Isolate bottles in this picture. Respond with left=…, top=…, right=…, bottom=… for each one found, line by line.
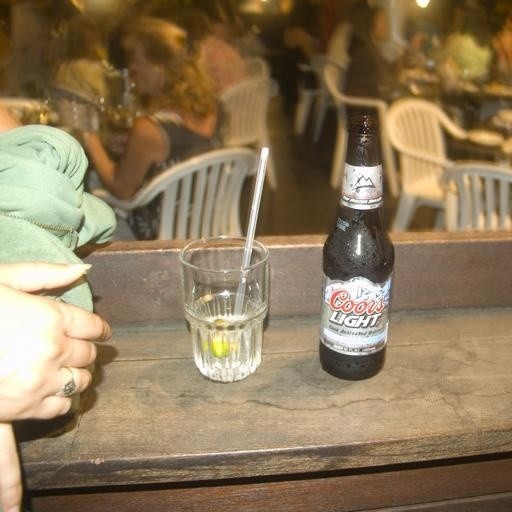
left=318, top=113, right=397, bottom=383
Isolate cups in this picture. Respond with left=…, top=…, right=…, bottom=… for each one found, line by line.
left=179, top=235, right=269, bottom=385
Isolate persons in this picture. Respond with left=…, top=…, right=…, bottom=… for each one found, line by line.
left=1, top=1, right=512, bottom=244
left=0, top=261, right=115, bottom=510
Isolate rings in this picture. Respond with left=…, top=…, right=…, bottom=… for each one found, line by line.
left=55, top=367, right=78, bottom=398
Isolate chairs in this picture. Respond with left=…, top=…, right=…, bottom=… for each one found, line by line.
left=1, top=96, right=54, bottom=126
left=380, top=96, right=479, bottom=241
left=439, top=162, right=510, bottom=239
left=89, top=145, right=266, bottom=239
left=292, top=20, right=510, bottom=189
left=214, top=56, right=283, bottom=191
